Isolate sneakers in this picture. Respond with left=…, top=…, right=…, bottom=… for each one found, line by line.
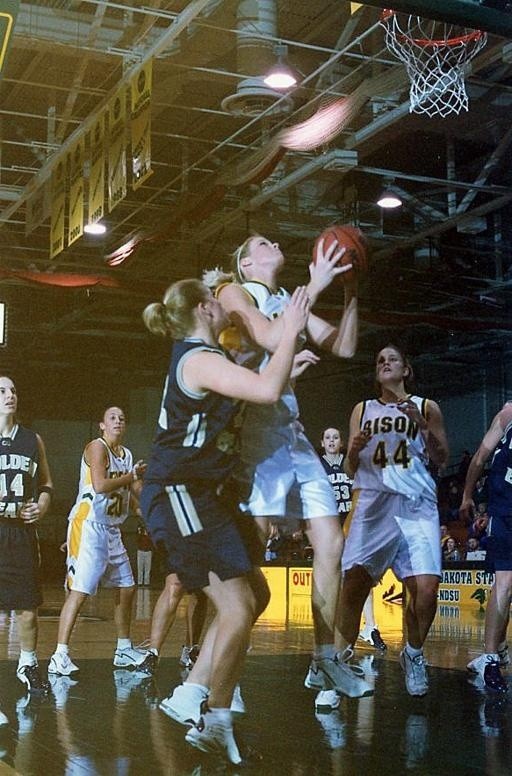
left=304, top=625, right=386, bottom=710
left=159, top=680, right=246, bottom=765
left=113, top=639, right=199, bottom=675
left=399, top=644, right=430, bottom=697
left=47, top=653, right=79, bottom=676
left=16, top=663, right=50, bottom=694
left=466, top=641, right=511, bottom=695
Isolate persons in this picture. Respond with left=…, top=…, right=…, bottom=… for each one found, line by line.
left=0, top=232, right=509, bottom=766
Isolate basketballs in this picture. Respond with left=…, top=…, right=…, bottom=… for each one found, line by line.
left=313, top=226, right=373, bottom=286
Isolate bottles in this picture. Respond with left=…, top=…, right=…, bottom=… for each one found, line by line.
left=454, top=549, right=459, bottom=561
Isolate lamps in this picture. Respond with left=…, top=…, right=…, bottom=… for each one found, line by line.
left=263, top=35, right=298, bottom=89
left=376, top=175, right=403, bottom=209
